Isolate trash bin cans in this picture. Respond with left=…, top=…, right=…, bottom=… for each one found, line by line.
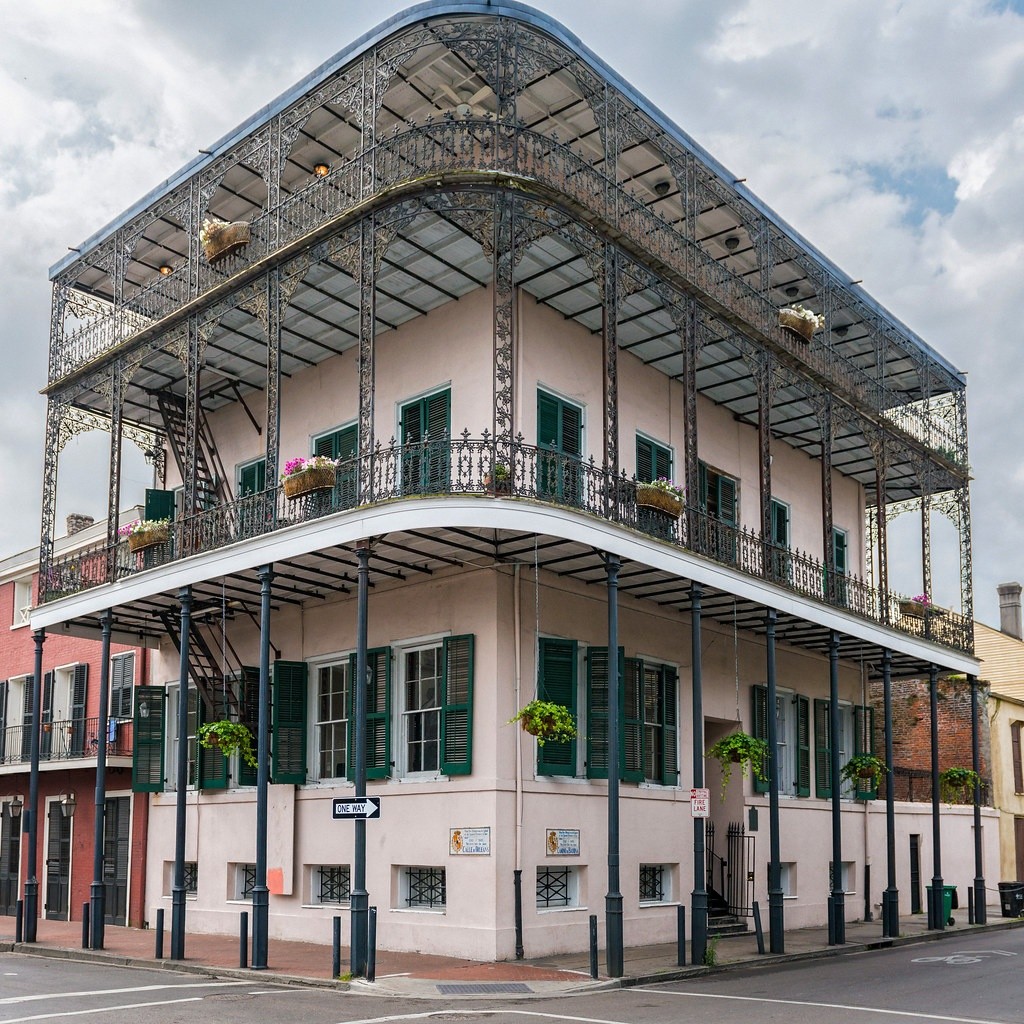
left=925, top=885, right=957, bottom=927
left=998, top=881, right=1024, bottom=917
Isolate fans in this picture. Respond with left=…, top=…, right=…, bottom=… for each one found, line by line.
left=430, top=82, right=496, bottom=119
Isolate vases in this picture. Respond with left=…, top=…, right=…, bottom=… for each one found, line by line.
left=203, top=221, right=250, bottom=265
left=899, top=600, right=926, bottom=619
left=283, top=468, right=337, bottom=499
left=127, top=528, right=167, bottom=553
left=779, top=308, right=813, bottom=344
left=636, top=484, right=682, bottom=519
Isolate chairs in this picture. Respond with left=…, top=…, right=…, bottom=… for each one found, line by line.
left=87, top=719, right=117, bottom=755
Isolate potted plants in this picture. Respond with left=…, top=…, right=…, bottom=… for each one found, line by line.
left=484, top=463, right=511, bottom=498
left=938, top=766, right=985, bottom=805
left=839, top=754, right=891, bottom=793
left=702, top=731, right=773, bottom=804
left=195, top=719, right=258, bottom=769
left=506, top=699, right=580, bottom=747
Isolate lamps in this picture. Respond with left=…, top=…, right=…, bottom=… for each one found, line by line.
left=159, top=265, right=173, bottom=275
left=649, top=177, right=850, bottom=339
left=313, top=163, right=330, bottom=177
left=6, top=789, right=24, bottom=818
left=59, top=787, right=78, bottom=818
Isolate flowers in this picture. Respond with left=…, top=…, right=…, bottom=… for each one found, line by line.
left=791, top=303, right=825, bottom=329
left=116, top=514, right=173, bottom=536
left=200, top=217, right=231, bottom=244
left=913, top=591, right=933, bottom=609
left=643, top=476, right=689, bottom=498
left=279, top=454, right=342, bottom=480
